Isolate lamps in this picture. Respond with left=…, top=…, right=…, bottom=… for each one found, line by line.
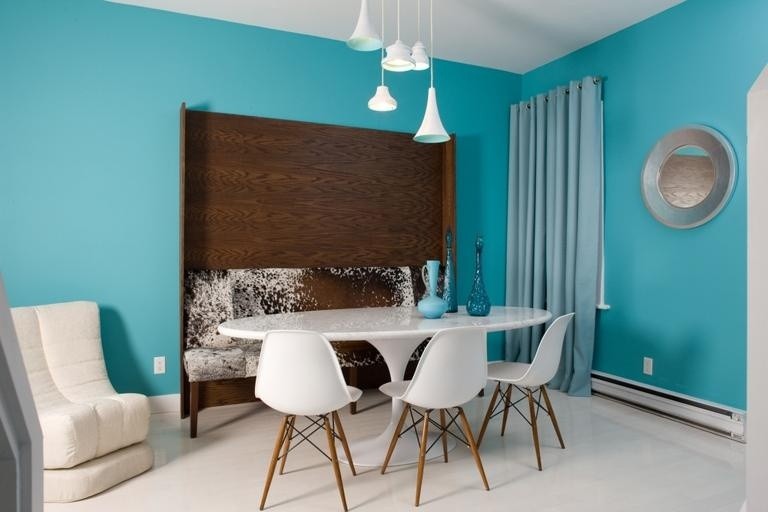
left=367, top=2, right=398, bottom=111
left=345, top=0, right=382, bottom=52
left=412, top=0, right=450, bottom=144
left=409, top=0, right=430, bottom=71
left=380, top=0, right=415, bottom=73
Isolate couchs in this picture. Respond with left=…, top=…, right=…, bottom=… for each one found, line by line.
left=1, top=298, right=155, bottom=505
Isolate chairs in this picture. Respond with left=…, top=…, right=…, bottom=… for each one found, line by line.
left=379, top=325, right=491, bottom=509
left=253, top=328, right=363, bottom=512
left=474, top=311, right=576, bottom=473
left=177, top=265, right=456, bottom=440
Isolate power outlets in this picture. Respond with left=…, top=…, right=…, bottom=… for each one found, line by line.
left=643, top=356, right=653, bottom=376
left=153, top=356, right=166, bottom=373
